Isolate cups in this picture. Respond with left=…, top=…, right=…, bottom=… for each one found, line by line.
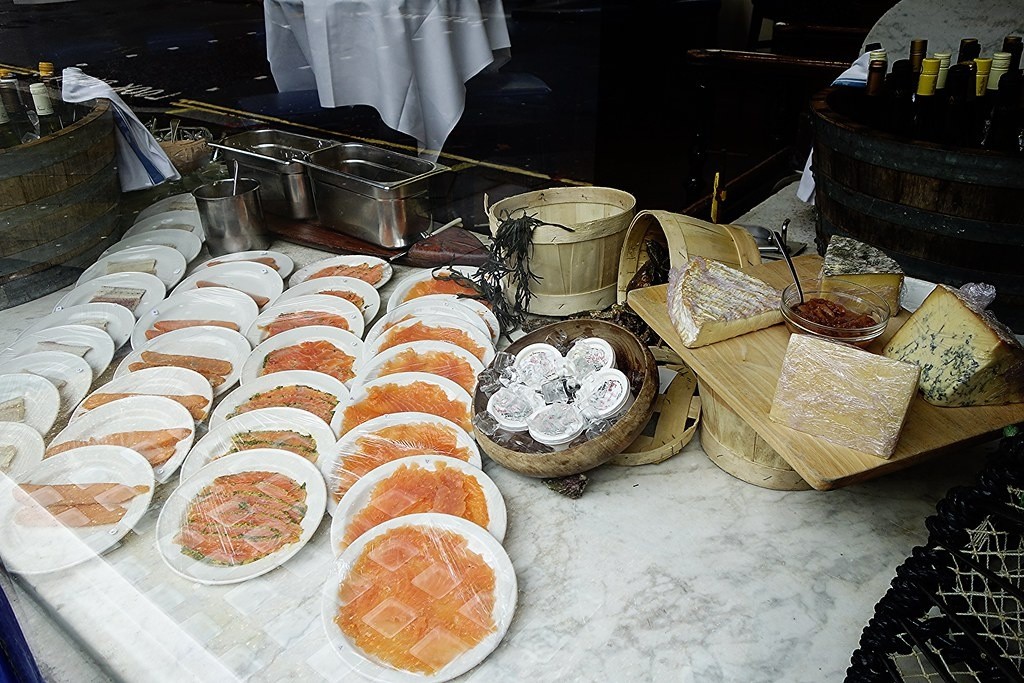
left=191, top=177, right=270, bottom=257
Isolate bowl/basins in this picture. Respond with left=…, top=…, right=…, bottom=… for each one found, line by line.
left=779, top=278, right=891, bottom=348
left=470, top=319, right=659, bottom=476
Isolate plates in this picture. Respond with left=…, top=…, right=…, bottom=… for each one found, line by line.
left=0, top=193, right=518, bottom=683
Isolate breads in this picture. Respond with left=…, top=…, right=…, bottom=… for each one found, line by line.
left=768, top=333, right=922, bottom=459
left=882, top=285, right=1024, bottom=406
left=666, top=255, right=786, bottom=348
left=819, top=235, right=904, bottom=316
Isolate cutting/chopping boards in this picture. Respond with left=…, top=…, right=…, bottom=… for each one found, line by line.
left=627, top=250, right=1024, bottom=490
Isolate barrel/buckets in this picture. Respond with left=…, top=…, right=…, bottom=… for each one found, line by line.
left=692, top=371, right=806, bottom=490
left=489, top=186, right=637, bottom=317
left=811, top=87, right=1024, bottom=330
left=617, top=209, right=762, bottom=303
left=0, top=98, right=135, bottom=311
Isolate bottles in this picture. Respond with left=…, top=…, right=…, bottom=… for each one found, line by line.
left=849, top=35, right=1024, bottom=158
left=0, top=62, right=65, bottom=148
